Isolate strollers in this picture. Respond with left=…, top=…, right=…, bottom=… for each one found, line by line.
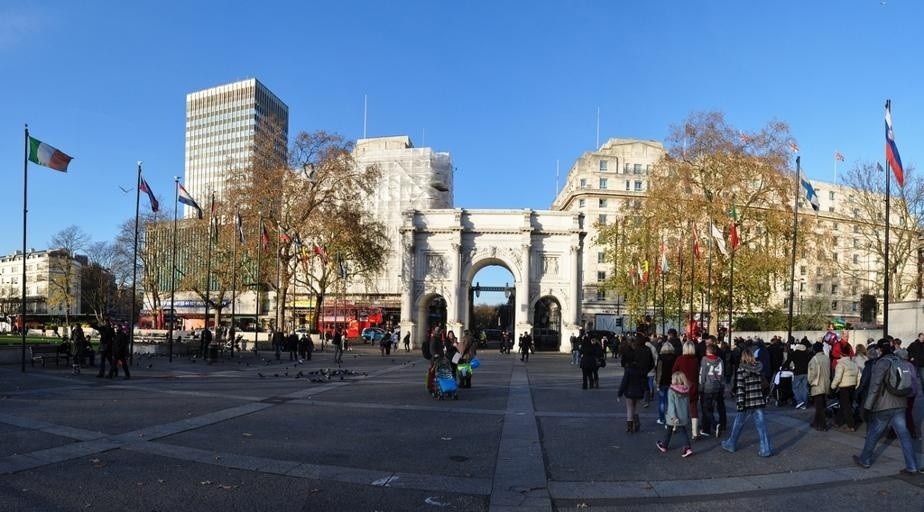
left=765, top=357, right=794, bottom=408
left=432, top=358, right=458, bottom=401
left=825, top=397, right=863, bottom=428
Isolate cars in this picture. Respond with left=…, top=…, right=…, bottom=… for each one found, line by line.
left=586, top=329, right=620, bottom=346
left=474, top=327, right=513, bottom=342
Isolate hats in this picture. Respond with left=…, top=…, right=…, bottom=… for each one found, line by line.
left=433, top=327, right=442, bottom=335
left=877, top=339, right=891, bottom=349
left=813, top=341, right=824, bottom=350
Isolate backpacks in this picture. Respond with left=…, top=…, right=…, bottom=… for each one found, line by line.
left=422, top=339, right=433, bottom=360
left=880, top=356, right=913, bottom=397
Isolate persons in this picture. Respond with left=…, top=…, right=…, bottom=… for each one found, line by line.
left=267, top=321, right=349, bottom=364
left=189, top=323, right=235, bottom=361
left=572, top=322, right=923, bottom=475
left=12, top=314, right=130, bottom=381
left=363, top=322, right=535, bottom=396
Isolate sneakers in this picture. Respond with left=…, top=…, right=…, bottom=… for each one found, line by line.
left=795, top=401, right=807, bottom=410
left=758, top=450, right=771, bottom=458
left=722, top=441, right=736, bottom=453
left=655, top=416, right=722, bottom=457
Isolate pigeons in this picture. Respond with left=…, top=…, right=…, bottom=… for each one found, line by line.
left=134, top=352, right=275, bottom=368
left=258, top=364, right=369, bottom=383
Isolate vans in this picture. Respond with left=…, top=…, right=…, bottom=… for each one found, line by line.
left=534, top=327, right=559, bottom=347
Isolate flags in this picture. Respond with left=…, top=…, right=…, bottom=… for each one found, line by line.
left=27, top=135, right=74, bottom=173
left=630, top=206, right=738, bottom=289
left=138, top=172, right=159, bottom=213
left=799, top=168, right=820, bottom=211
left=884, top=106, right=904, bottom=190
left=235, top=211, right=244, bottom=243
left=211, top=200, right=219, bottom=245
left=836, top=152, right=846, bottom=164
left=260, top=217, right=348, bottom=279
left=176, top=181, right=202, bottom=220
left=787, top=140, right=799, bottom=154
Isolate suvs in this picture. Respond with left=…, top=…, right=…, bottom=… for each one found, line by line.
left=685, top=312, right=734, bottom=341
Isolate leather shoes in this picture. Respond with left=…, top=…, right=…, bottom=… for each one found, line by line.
left=900, top=468, right=918, bottom=475
left=853, top=455, right=870, bottom=469
left=809, top=422, right=856, bottom=431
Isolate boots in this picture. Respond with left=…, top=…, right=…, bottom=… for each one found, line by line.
left=625, top=421, right=633, bottom=432
left=75, top=364, right=80, bottom=375
left=634, top=414, right=641, bottom=431
left=71, top=365, right=75, bottom=375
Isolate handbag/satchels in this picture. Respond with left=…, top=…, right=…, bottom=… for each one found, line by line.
left=437, top=376, right=457, bottom=393
left=451, top=352, right=462, bottom=364
left=808, top=358, right=820, bottom=387
left=715, top=385, right=727, bottom=400
left=600, top=359, right=606, bottom=367
left=760, top=373, right=770, bottom=395
left=825, top=397, right=840, bottom=414
left=458, top=357, right=464, bottom=364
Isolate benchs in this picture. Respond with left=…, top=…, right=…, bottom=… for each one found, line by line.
left=157, top=342, right=187, bottom=357
left=28, top=344, right=75, bottom=367
